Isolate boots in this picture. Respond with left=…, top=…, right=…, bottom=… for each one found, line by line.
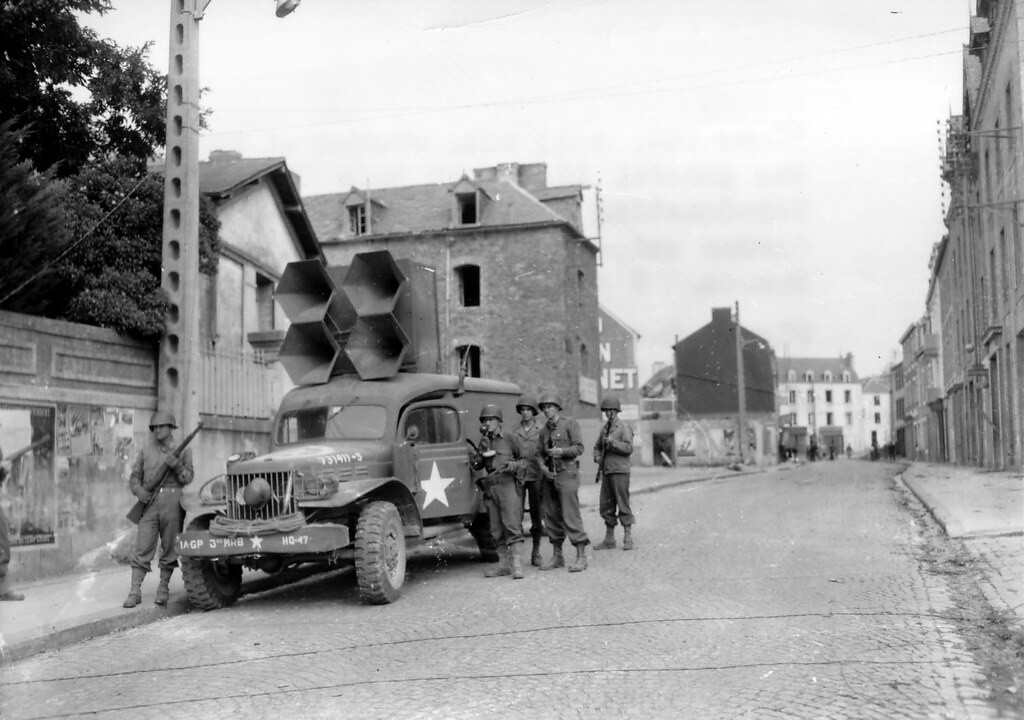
left=540, top=542, right=565, bottom=570
left=592, top=525, right=617, bottom=550
left=624, top=524, right=634, bottom=550
left=568, top=543, right=588, bottom=572
left=485, top=544, right=512, bottom=576
left=510, top=540, right=524, bottom=579
left=531, top=534, right=543, bottom=566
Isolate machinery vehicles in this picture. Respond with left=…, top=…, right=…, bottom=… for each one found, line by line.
left=173, top=249, right=527, bottom=611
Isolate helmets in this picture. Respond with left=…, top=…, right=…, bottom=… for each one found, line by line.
left=148, top=411, right=177, bottom=431
left=516, top=397, right=540, bottom=416
left=601, top=396, right=621, bottom=412
left=479, top=405, right=504, bottom=424
left=244, top=478, right=271, bottom=511
left=538, top=394, right=563, bottom=412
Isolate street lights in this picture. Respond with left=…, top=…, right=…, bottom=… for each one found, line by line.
left=156, top=0, right=304, bottom=506
left=735, top=300, right=766, bottom=463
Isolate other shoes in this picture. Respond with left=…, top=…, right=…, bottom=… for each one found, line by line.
left=154, top=585, right=169, bottom=603
left=0, top=590, right=25, bottom=601
left=122, top=584, right=141, bottom=607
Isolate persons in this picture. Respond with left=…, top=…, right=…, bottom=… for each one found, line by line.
left=0, top=449, right=24, bottom=602
left=507, top=395, right=591, bottom=571
left=593, top=395, right=635, bottom=550
left=474, top=402, right=527, bottom=580
left=122, top=411, right=194, bottom=608
left=777, top=441, right=898, bottom=462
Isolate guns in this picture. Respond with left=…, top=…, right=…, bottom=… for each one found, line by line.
left=596, top=411, right=614, bottom=483
left=126, top=419, right=205, bottom=526
left=488, top=454, right=516, bottom=477
left=546, top=436, right=563, bottom=508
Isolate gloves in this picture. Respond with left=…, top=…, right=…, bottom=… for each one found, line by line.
left=166, top=454, right=183, bottom=471
left=136, top=487, right=152, bottom=504
left=503, top=461, right=517, bottom=473
left=543, top=466, right=558, bottom=480
left=548, top=447, right=563, bottom=458
